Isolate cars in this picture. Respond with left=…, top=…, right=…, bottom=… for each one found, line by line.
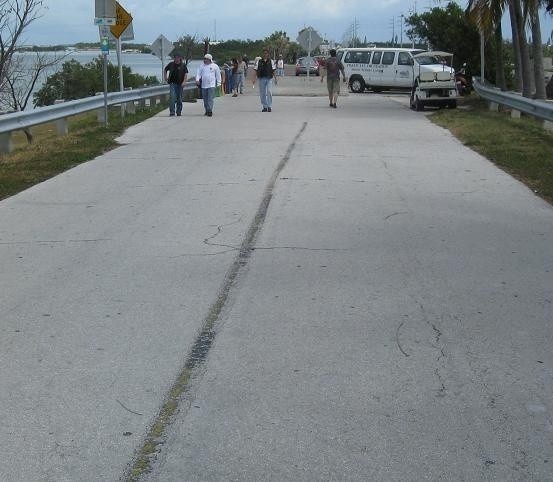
left=408, top=50, right=458, bottom=111
left=295, top=56, right=320, bottom=76
left=314, top=55, right=326, bottom=66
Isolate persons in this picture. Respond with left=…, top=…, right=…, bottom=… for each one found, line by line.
left=163, top=53, right=189, bottom=117
left=249, top=49, right=277, bottom=113
left=319, top=49, right=346, bottom=109
left=222, top=53, right=262, bottom=97
left=269, top=54, right=285, bottom=81
left=194, top=54, right=221, bottom=117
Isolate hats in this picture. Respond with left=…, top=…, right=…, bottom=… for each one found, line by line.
left=204, top=54, right=212, bottom=61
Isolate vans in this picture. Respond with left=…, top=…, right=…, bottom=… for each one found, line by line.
left=335, top=47, right=426, bottom=93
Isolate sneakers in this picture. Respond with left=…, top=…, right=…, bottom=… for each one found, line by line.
left=329, top=103, right=336, bottom=108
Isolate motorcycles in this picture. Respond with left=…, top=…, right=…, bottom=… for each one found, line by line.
left=453, top=62, right=472, bottom=96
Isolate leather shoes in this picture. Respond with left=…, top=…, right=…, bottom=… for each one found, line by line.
left=170, top=113, right=181, bottom=116
left=262, top=107, right=271, bottom=112
left=205, top=111, right=212, bottom=116
